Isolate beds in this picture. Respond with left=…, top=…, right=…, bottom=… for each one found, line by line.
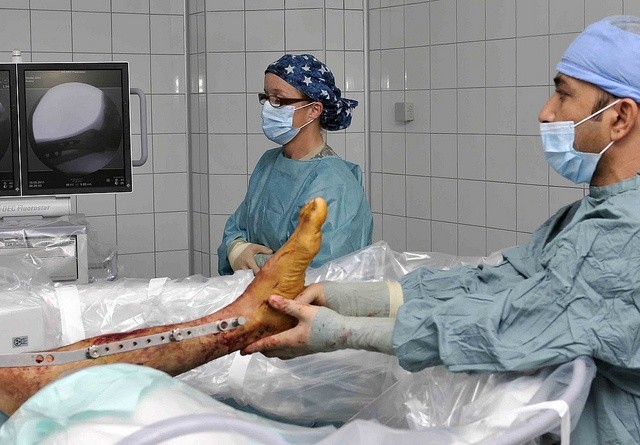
left=2, top=248, right=595, bottom=445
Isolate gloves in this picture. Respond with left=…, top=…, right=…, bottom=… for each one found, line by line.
left=240, top=294, right=396, bottom=360
left=293, top=279, right=404, bottom=319
left=226, top=237, right=273, bottom=276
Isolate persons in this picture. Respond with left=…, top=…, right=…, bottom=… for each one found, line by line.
left=0, top=197, right=327, bottom=417
left=217, top=53, right=373, bottom=279
left=241, top=15, right=640, bottom=444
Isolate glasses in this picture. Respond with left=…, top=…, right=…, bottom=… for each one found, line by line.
left=257, top=91, right=310, bottom=107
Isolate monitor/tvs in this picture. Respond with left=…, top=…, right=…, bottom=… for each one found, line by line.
left=17, top=60, right=134, bottom=198
left=0, top=62, right=21, bottom=199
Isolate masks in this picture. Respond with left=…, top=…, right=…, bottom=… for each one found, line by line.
left=260, top=99, right=320, bottom=145
left=538, top=99, right=623, bottom=185
